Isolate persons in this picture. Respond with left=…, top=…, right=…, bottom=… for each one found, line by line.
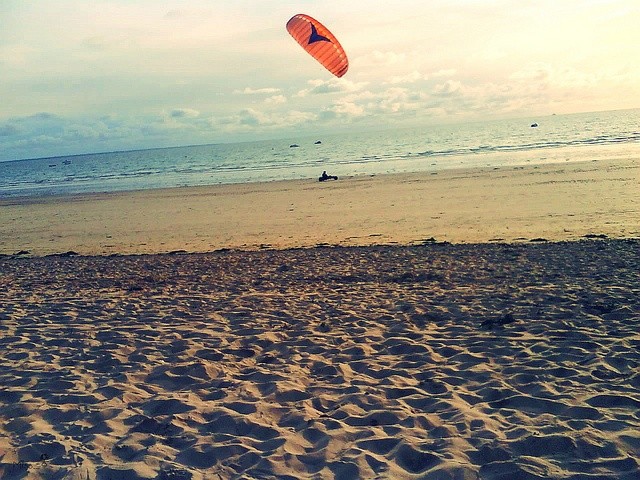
left=322, top=170, right=328, bottom=177
